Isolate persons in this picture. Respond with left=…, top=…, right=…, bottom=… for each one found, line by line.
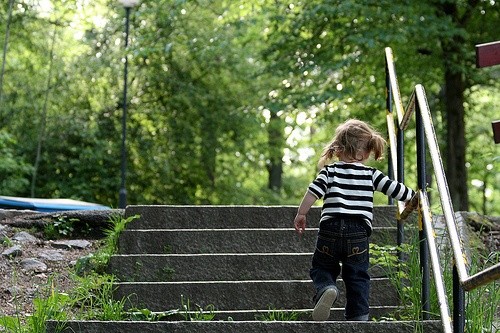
left=294, top=119, right=419, bottom=321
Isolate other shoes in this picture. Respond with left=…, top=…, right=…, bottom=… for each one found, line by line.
left=312, top=288, right=337, bottom=320
